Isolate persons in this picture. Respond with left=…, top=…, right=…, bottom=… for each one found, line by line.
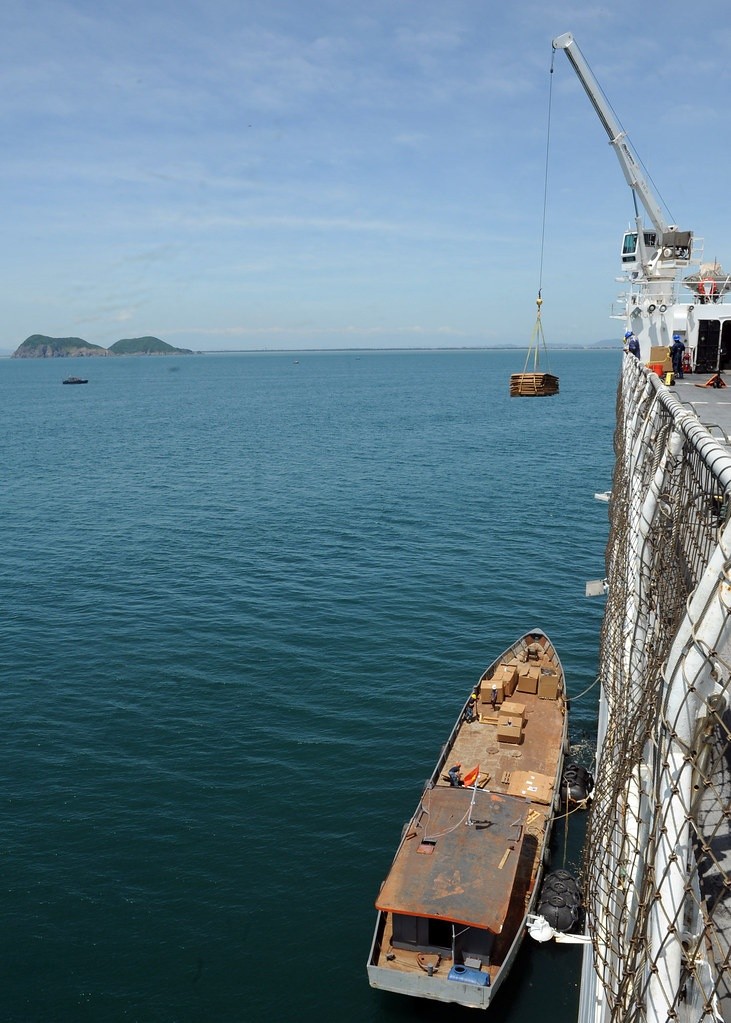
left=669, top=334, right=685, bottom=379
left=448, top=763, right=462, bottom=787
left=623, top=331, right=641, bottom=361
left=465, top=694, right=477, bottom=723
left=489, top=685, right=498, bottom=709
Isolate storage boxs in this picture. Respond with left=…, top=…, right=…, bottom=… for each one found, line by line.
left=480, top=680, right=504, bottom=704
left=650, top=346, right=673, bottom=372
left=496, top=716, right=523, bottom=745
left=491, top=672, right=515, bottom=697
left=498, top=701, right=526, bottom=724
left=518, top=665, right=539, bottom=693
left=538, top=667, right=560, bottom=700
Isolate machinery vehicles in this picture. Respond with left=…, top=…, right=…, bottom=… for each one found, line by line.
left=547, top=30, right=693, bottom=286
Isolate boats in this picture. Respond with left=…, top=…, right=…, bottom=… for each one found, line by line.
left=363, top=625, right=568, bottom=1012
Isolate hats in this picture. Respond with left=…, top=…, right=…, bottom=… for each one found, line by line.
left=492, top=684, right=497, bottom=689
left=472, top=694, right=476, bottom=699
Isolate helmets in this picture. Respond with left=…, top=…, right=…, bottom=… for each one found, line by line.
left=674, top=335, right=680, bottom=341
left=625, top=332, right=633, bottom=338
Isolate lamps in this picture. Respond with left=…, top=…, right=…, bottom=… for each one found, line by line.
left=594, top=491, right=612, bottom=501
left=585, top=578, right=609, bottom=598
